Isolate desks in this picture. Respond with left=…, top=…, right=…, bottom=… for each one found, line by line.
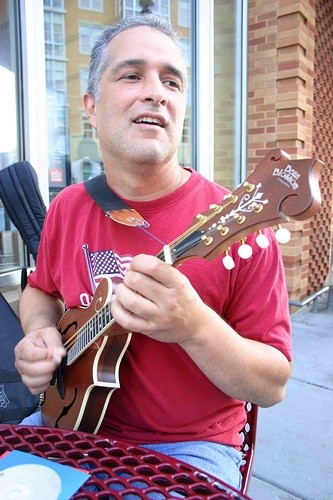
left=0, top=423, right=251, bottom=500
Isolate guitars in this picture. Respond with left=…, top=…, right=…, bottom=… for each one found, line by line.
left=37, top=148, right=325, bottom=435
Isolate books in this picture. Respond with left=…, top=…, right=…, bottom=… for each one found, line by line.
left=0, top=450, right=91, bottom=500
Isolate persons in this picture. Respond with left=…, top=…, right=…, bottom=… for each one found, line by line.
left=0, top=17, right=294, bottom=500
